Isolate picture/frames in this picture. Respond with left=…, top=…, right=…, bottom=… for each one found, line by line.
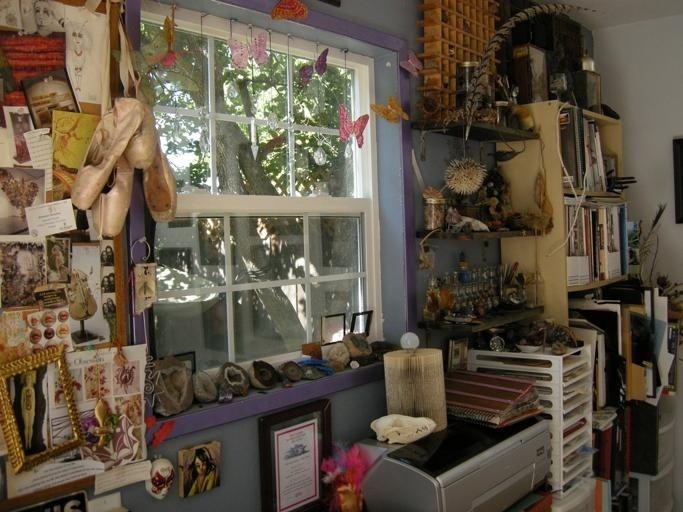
left=0, top=344, right=84, bottom=476
left=256, top=397, right=333, bottom=512
left=158, top=350, right=197, bottom=376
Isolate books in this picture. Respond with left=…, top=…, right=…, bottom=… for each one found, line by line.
left=559, top=107, right=633, bottom=288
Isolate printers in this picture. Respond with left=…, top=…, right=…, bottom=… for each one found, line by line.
left=355, top=415, right=552, bottom=512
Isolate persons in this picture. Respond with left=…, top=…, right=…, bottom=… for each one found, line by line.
left=188, top=448, right=217, bottom=497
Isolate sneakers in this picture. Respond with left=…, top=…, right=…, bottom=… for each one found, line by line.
left=69, top=68, right=176, bottom=240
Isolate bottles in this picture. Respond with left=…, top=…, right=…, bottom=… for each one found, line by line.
left=422, top=196, right=447, bottom=231
left=491, top=100, right=509, bottom=129
left=455, top=59, right=480, bottom=91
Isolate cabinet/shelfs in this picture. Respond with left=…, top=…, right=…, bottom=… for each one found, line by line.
left=409, top=119, right=546, bottom=335
left=467, top=343, right=594, bottom=500
left=494, top=99, right=629, bottom=342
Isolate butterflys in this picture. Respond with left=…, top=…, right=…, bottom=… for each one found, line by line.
left=337, top=104, right=369, bottom=149
left=228, top=30, right=269, bottom=71
left=298, top=47, right=330, bottom=87
left=369, top=96, right=412, bottom=124
left=397, top=48, right=424, bottom=76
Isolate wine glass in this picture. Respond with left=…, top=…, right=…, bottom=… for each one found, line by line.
left=548, top=72, right=570, bottom=103
